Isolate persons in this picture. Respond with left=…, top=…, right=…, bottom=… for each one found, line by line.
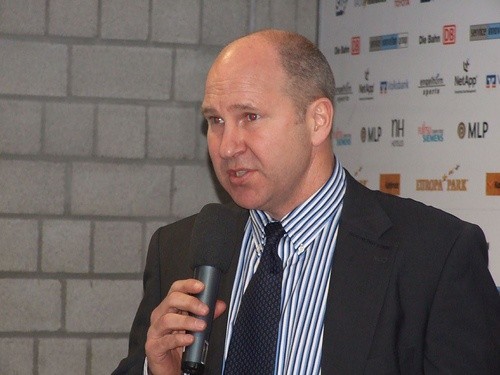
left=105, top=28, right=499, bottom=374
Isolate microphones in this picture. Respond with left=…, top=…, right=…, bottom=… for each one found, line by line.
left=180, top=202, right=240, bottom=375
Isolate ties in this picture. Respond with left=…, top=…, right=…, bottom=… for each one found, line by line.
left=222, top=222, right=287, bottom=375
